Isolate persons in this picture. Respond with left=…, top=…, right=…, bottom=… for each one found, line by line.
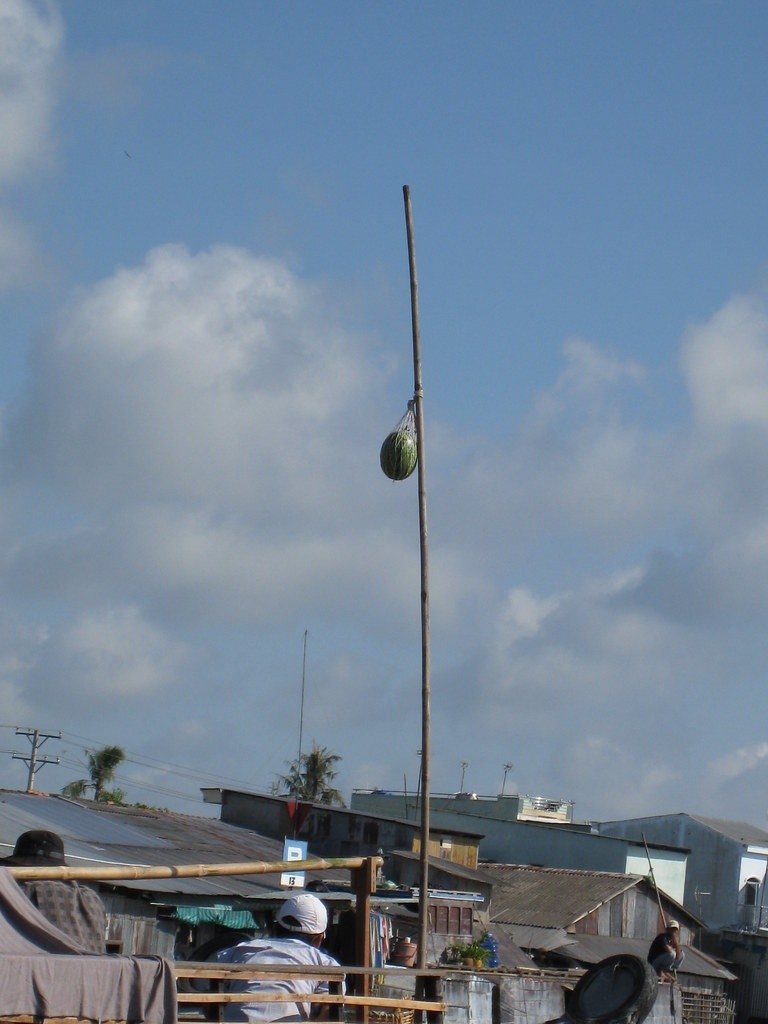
left=646, top=919, right=685, bottom=982
left=197, top=894, right=348, bottom=1022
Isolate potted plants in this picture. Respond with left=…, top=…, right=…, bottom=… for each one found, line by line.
left=446, top=928, right=492, bottom=972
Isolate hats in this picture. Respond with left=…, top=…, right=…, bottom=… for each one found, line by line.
left=666, top=920, right=679, bottom=931
left=0, top=830, right=67, bottom=866
left=275, top=894, right=328, bottom=939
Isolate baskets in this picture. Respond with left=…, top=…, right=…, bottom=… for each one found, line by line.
left=348, top=994, right=414, bottom=1024
